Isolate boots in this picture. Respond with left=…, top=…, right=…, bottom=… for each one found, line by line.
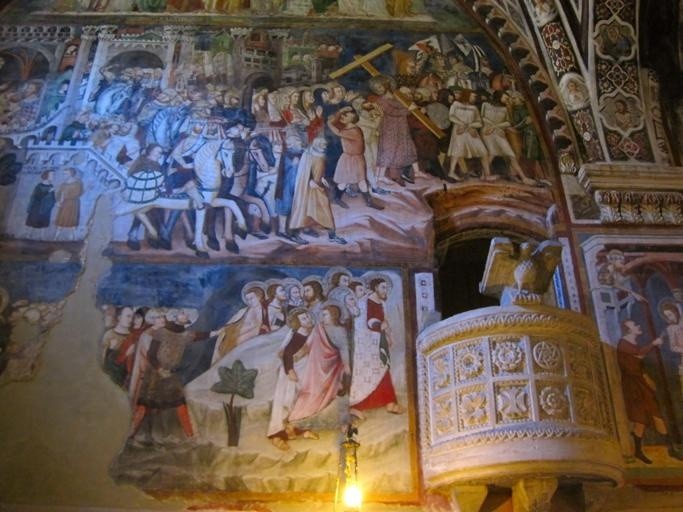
left=362, top=191, right=386, bottom=211
left=631, top=433, right=654, bottom=464
left=333, top=188, right=351, bottom=209
left=659, top=434, right=683, bottom=461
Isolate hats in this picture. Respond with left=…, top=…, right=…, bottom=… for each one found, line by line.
left=417, top=87, right=431, bottom=100
left=310, top=137, right=327, bottom=155
left=126, top=306, right=199, bottom=329
left=241, top=267, right=394, bottom=328
left=391, top=50, right=408, bottom=66
left=210, top=82, right=346, bottom=103
left=491, top=76, right=501, bottom=89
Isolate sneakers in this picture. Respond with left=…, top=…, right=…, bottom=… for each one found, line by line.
left=304, top=228, right=319, bottom=238
left=328, top=231, right=347, bottom=245
left=270, top=401, right=407, bottom=452
left=447, top=169, right=553, bottom=190
left=373, top=173, right=432, bottom=196
